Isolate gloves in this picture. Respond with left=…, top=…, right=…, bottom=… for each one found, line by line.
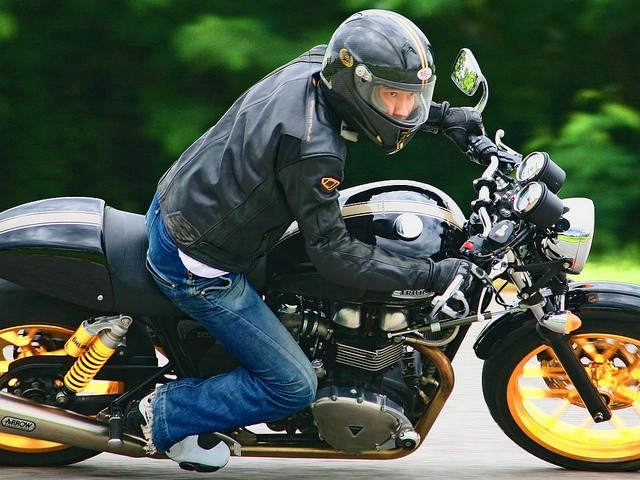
left=428, top=257, right=472, bottom=298
left=440, top=102, right=485, bottom=153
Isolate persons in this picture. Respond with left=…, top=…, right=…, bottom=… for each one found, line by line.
left=138, top=10, right=492, bottom=474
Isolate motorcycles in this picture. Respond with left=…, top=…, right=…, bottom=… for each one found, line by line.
left=1, top=44, right=639, bottom=474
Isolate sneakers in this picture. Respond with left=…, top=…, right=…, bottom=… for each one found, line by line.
left=124, top=395, right=230, bottom=472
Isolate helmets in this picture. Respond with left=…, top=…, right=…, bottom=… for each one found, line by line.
left=319, top=9, right=436, bottom=156
